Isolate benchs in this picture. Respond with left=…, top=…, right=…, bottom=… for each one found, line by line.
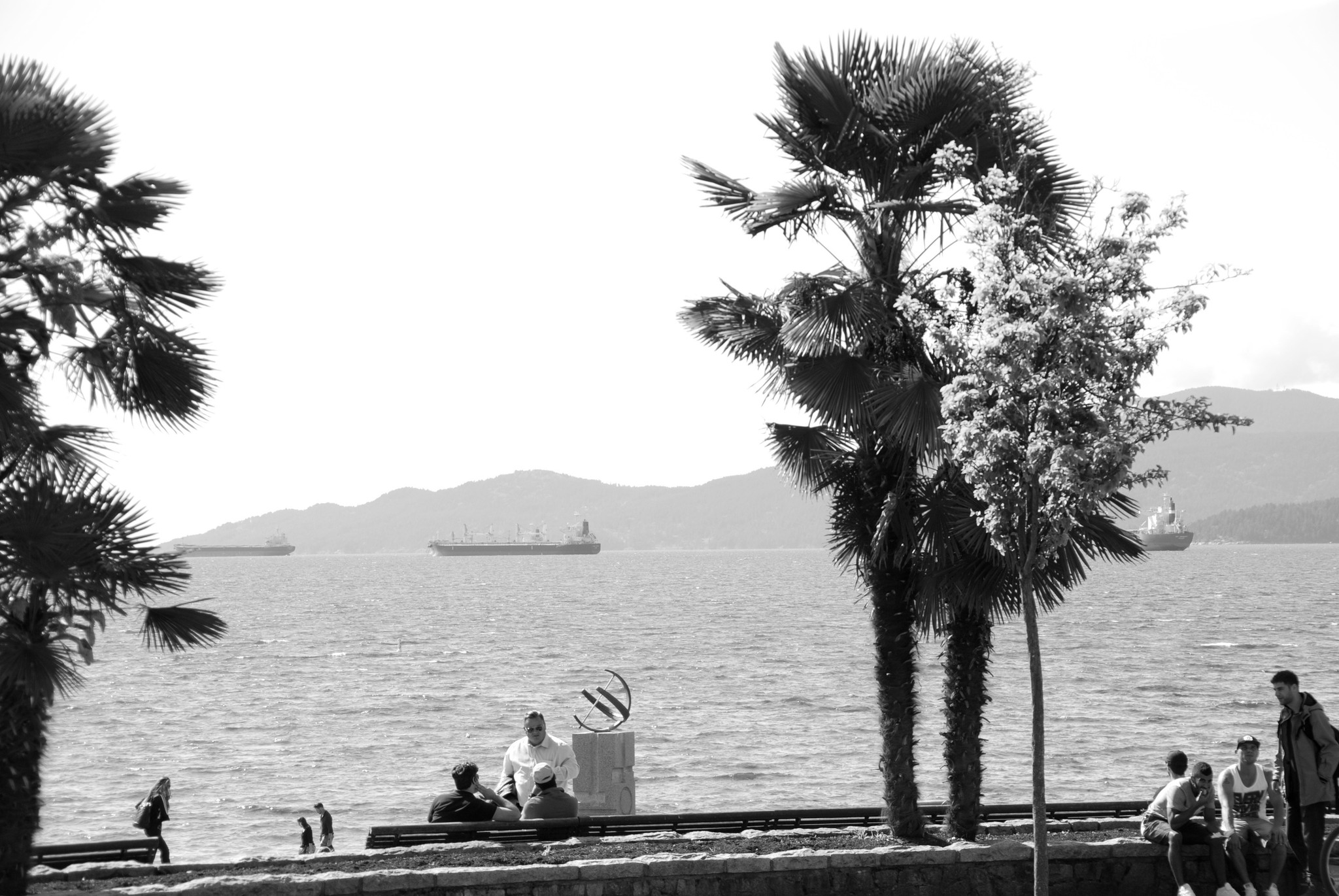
left=26, top=835, right=158, bottom=871
left=365, top=799, right=1157, bottom=838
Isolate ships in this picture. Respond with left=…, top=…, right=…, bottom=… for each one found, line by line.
left=171, top=532, right=295, bottom=556
left=1124, top=492, right=1194, bottom=552
left=429, top=520, right=600, bottom=556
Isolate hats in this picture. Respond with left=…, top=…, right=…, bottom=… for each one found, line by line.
left=1237, top=735, right=1260, bottom=751
left=1165, top=751, right=1187, bottom=766
left=533, top=762, right=554, bottom=784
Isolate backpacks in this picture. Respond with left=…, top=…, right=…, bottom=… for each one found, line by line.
left=133, top=796, right=154, bottom=829
left=1294, top=714, right=1339, bottom=798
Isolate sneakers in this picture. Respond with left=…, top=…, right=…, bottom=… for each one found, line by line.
left=1216, top=882, right=1241, bottom=896
left=1266, top=883, right=1279, bottom=896
left=1243, top=882, right=1257, bottom=896
left=1177, top=883, right=1196, bottom=896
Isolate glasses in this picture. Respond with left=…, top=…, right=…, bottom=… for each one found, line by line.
left=525, top=725, right=544, bottom=733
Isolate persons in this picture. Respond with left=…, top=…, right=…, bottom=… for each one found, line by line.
left=1270, top=669, right=1339, bottom=896
left=135, top=776, right=172, bottom=865
left=499, top=708, right=579, bottom=814
left=520, top=761, right=580, bottom=825
left=296, top=816, right=316, bottom=854
left=313, top=801, right=338, bottom=853
left=1140, top=762, right=1242, bottom=896
left=1215, top=733, right=1290, bottom=896
left=426, top=760, right=522, bottom=841
left=316, top=846, right=332, bottom=854
left=1152, top=749, right=1190, bottom=802
